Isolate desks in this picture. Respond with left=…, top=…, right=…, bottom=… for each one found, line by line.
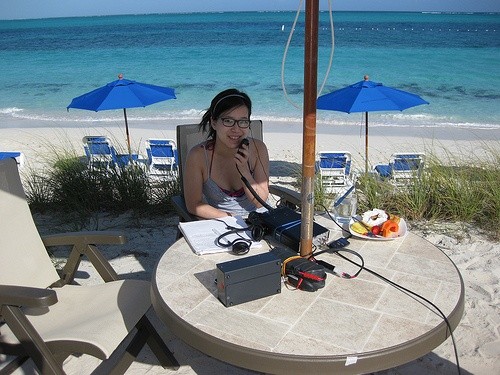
left=150, top=217, right=465, bottom=375
left=118, top=153, right=139, bottom=165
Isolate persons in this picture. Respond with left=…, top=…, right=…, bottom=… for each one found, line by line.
left=183, top=89, right=272, bottom=219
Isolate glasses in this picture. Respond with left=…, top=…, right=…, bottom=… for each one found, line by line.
left=216, top=117, right=251, bottom=128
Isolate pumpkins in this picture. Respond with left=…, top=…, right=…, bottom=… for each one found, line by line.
left=381, top=220, right=398, bottom=237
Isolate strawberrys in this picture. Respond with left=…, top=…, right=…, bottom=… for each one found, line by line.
left=372, top=226, right=380, bottom=235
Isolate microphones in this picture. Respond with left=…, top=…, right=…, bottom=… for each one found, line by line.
left=236, top=139, right=249, bottom=163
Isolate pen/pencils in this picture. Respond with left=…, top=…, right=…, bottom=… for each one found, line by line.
left=213, top=228, right=232, bottom=245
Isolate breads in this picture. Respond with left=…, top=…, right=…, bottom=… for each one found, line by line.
left=351, top=223, right=369, bottom=234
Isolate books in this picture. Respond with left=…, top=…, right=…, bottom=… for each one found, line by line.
left=178, top=215, right=262, bottom=255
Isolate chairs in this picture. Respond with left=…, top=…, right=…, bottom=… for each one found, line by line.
left=316, top=150, right=354, bottom=189
left=145, top=138, right=179, bottom=175
left=169, top=120, right=304, bottom=243
left=0, top=154, right=183, bottom=375
left=374, top=151, right=429, bottom=188
left=81, top=135, right=123, bottom=173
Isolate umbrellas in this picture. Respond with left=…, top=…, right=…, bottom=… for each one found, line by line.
left=317, top=75, right=430, bottom=173
left=66, top=74, right=177, bottom=165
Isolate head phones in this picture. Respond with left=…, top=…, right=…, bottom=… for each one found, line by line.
left=218, top=222, right=264, bottom=254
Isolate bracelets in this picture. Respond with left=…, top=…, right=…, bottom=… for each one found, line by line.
left=226, top=212, right=232, bottom=217
